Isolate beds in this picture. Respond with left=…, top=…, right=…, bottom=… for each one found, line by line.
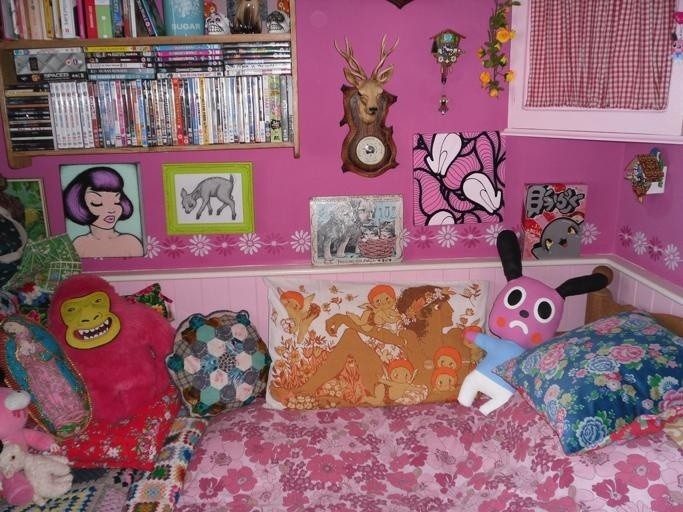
left=0, top=266, right=683, bottom=512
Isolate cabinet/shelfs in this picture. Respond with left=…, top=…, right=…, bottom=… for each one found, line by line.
left=1, top=0, right=300, bottom=169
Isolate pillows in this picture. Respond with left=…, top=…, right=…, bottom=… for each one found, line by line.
left=262, top=279, right=490, bottom=413
left=494, top=307, right=683, bottom=454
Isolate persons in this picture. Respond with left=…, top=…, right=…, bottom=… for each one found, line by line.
left=285, top=285, right=471, bottom=404
left=65, top=166, right=143, bottom=256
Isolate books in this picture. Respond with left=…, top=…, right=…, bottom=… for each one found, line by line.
left=1, top=1, right=296, bottom=151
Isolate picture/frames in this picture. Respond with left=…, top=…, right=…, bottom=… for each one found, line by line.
left=310, top=194, right=405, bottom=265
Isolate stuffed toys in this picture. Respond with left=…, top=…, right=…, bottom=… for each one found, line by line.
left=0, top=274, right=177, bottom=507
left=0, top=273, right=176, bottom=506
left=457, top=229, right=608, bottom=415
